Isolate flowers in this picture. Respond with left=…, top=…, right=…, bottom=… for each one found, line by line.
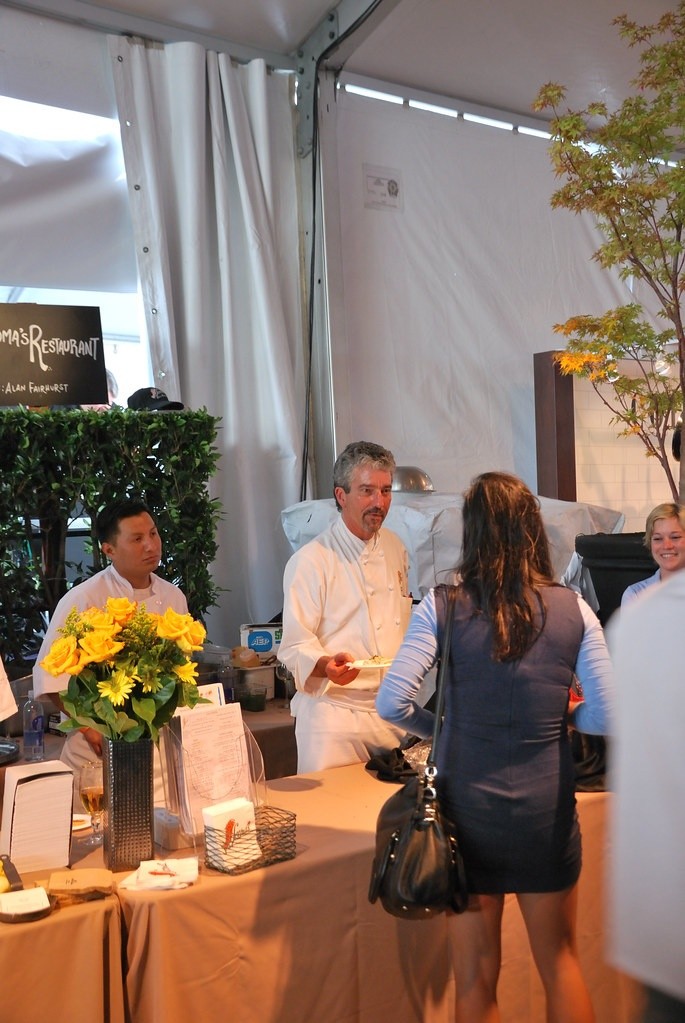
left=39, top=600, right=210, bottom=745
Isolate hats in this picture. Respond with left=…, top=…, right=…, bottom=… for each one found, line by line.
left=128, top=387, right=184, bottom=411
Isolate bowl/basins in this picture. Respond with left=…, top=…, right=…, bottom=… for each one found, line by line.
left=390, top=466, right=436, bottom=493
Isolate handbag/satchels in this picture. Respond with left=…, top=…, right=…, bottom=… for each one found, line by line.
left=368, top=768, right=468, bottom=921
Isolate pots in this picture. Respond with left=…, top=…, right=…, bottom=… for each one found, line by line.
left=1, top=853, right=57, bottom=924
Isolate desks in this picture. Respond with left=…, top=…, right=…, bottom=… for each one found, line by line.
left=0, top=868, right=123, bottom=1023
left=70, top=760, right=645, bottom=1023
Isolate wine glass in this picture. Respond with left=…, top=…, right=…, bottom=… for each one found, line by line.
left=276, top=658, right=294, bottom=710
left=78, top=761, right=106, bottom=846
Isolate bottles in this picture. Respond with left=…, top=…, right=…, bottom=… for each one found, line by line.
left=22, top=690, right=46, bottom=761
left=217, top=655, right=235, bottom=700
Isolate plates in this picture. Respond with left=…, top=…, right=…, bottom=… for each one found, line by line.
left=345, top=660, right=393, bottom=670
left=72, top=813, right=92, bottom=831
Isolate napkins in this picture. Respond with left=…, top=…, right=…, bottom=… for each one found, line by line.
left=122, top=797, right=262, bottom=891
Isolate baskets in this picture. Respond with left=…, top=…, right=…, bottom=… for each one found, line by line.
left=204, top=806, right=297, bottom=876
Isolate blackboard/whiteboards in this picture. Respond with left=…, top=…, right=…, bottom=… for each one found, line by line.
left=0, top=302, right=109, bottom=406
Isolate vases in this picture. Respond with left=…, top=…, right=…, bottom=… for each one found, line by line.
left=103, top=732, right=157, bottom=872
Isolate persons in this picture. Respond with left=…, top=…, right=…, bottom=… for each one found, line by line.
left=612, top=569, right=685, bottom=1023
left=376, top=471, right=612, bottom=1023
left=622, top=503, right=685, bottom=604
left=32, top=499, right=185, bottom=816
left=277, top=441, right=417, bottom=774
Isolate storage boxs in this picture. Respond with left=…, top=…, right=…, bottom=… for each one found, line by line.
left=240, top=621, right=282, bottom=655
